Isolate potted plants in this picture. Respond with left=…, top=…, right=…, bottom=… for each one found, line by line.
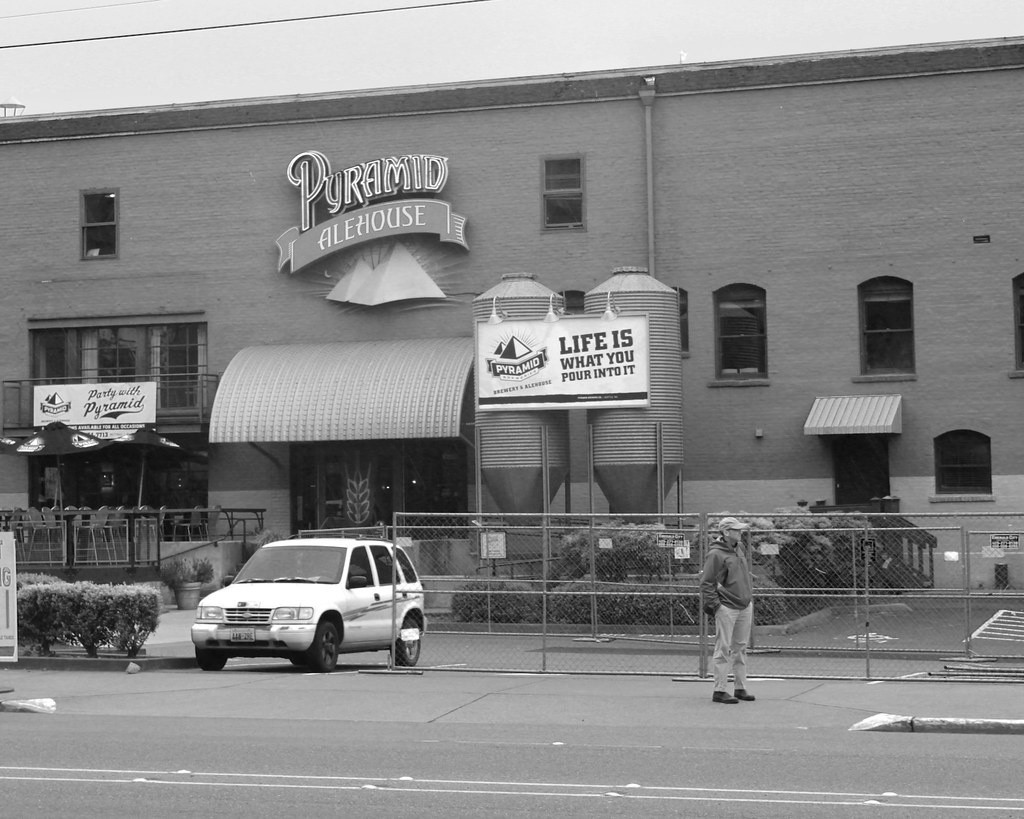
left=159, top=555, right=215, bottom=610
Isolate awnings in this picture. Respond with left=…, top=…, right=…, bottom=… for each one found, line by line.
left=804, top=394, right=902, bottom=435
left=208, top=337, right=475, bottom=443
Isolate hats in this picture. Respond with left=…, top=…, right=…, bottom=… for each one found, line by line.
left=718, top=517, right=748, bottom=531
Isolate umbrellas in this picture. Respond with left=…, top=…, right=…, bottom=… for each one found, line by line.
left=109, top=427, right=188, bottom=508
left=0, top=422, right=114, bottom=519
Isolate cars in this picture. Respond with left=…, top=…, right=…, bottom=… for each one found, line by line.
left=188, top=539, right=427, bottom=670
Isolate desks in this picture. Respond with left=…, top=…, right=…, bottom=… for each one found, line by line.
left=0, top=507, right=267, bottom=565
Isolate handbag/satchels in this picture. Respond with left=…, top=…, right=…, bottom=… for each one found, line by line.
left=703, top=602, right=715, bottom=616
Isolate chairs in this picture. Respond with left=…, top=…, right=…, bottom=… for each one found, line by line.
left=1, top=502, right=223, bottom=566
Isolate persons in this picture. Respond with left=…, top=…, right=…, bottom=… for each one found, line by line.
left=699, top=517, right=755, bottom=704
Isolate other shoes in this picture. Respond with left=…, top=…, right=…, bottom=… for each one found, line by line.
left=712, top=691, right=738, bottom=703
left=734, top=689, right=755, bottom=700
